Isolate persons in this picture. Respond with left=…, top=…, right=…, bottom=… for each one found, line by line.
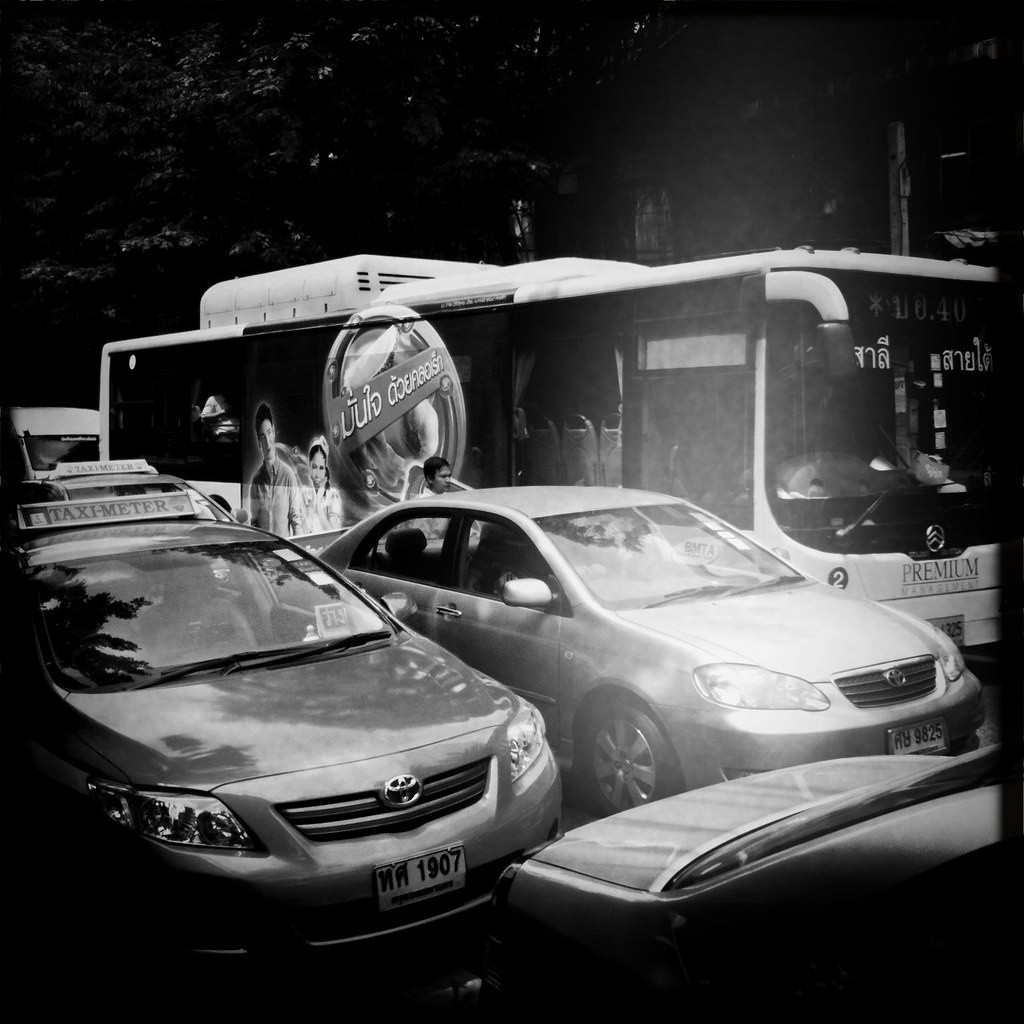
left=412, top=457, right=453, bottom=539
left=300, top=434, right=343, bottom=535
left=250, top=403, right=303, bottom=538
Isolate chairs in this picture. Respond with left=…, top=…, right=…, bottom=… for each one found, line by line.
left=383, top=523, right=693, bottom=606
left=523, top=410, right=622, bottom=495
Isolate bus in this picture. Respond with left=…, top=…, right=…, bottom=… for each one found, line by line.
left=100, top=244, right=1007, bottom=654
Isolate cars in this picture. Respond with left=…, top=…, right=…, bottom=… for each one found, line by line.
left=304, top=488, right=991, bottom=819
left=4, top=516, right=557, bottom=976
left=4, top=401, right=281, bottom=626
left=488, top=742, right=1000, bottom=1009
